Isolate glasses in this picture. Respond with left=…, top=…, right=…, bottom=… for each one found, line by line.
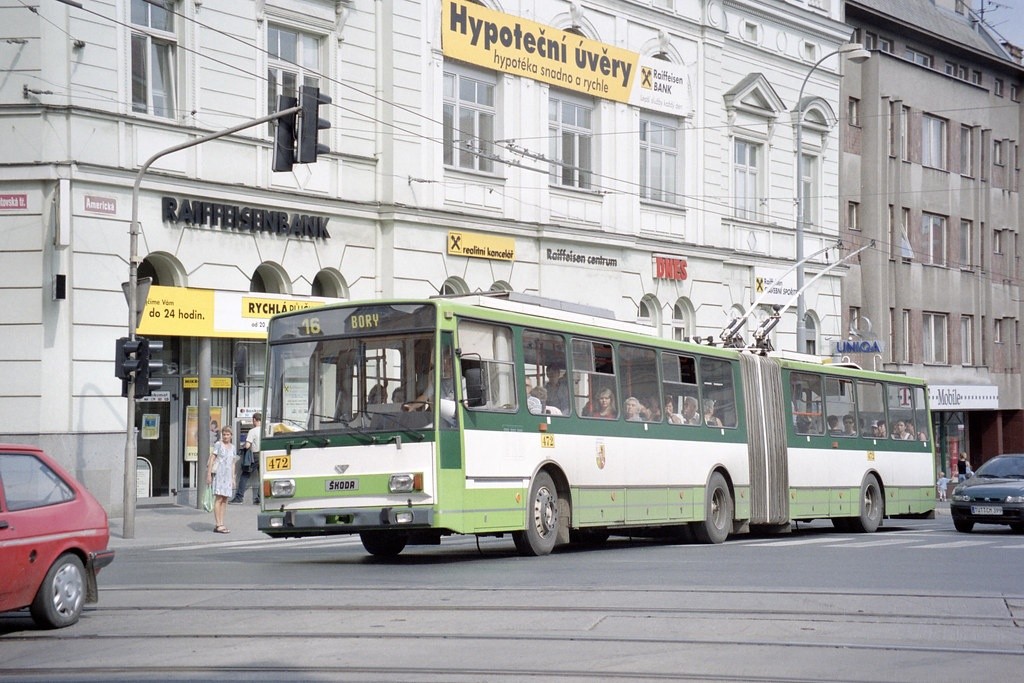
left=844, top=421, right=853, bottom=424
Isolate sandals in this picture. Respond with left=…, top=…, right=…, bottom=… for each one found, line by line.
left=214, top=524, right=230, bottom=532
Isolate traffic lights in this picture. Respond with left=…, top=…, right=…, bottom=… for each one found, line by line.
left=119, top=339, right=164, bottom=398
left=270, top=92, right=299, bottom=174
left=114, top=337, right=144, bottom=382
left=294, top=85, right=333, bottom=165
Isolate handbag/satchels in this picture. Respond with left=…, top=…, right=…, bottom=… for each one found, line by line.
left=206, top=440, right=221, bottom=473
left=201, top=483, right=214, bottom=513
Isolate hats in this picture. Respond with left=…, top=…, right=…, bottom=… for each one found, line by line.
left=871, top=419, right=878, bottom=427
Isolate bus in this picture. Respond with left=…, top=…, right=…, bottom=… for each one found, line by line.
left=234, top=242, right=938, bottom=560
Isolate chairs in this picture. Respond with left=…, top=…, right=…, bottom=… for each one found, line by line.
left=545, top=405, right=920, bottom=441
left=463, top=369, right=482, bottom=408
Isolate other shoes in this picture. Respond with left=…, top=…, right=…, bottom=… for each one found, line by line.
left=940, top=498, right=942, bottom=501
left=228, top=497, right=243, bottom=504
left=944, top=499, right=947, bottom=501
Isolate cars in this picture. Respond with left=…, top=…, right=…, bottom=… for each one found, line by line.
left=0, top=442, right=115, bottom=630
left=949, top=453, right=1024, bottom=534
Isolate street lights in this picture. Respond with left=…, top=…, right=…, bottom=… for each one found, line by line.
left=793, top=42, right=872, bottom=353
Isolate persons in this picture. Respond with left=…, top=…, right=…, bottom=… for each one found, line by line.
left=207, top=426, right=241, bottom=533
left=581, top=387, right=616, bottom=418
left=965, top=473, right=972, bottom=479
left=871, top=419, right=886, bottom=437
left=228, top=413, right=261, bottom=505
left=843, top=414, right=856, bottom=436
left=401, top=345, right=469, bottom=412
left=957, top=452, right=971, bottom=483
left=368, top=384, right=388, bottom=404
left=524, top=377, right=542, bottom=414
left=392, top=387, right=405, bottom=403
left=544, top=363, right=569, bottom=414
left=857, top=417, right=864, bottom=436
left=937, top=471, right=954, bottom=502
left=559, top=364, right=581, bottom=414
left=892, top=418, right=926, bottom=441
left=702, top=399, right=723, bottom=427
left=663, top=395, right=700, bottom=423
left=828, top=415, right=839, bottom=429
left=797, top=413, right=818, bottom=435
left=622, top=394, right=661, bottom=422
